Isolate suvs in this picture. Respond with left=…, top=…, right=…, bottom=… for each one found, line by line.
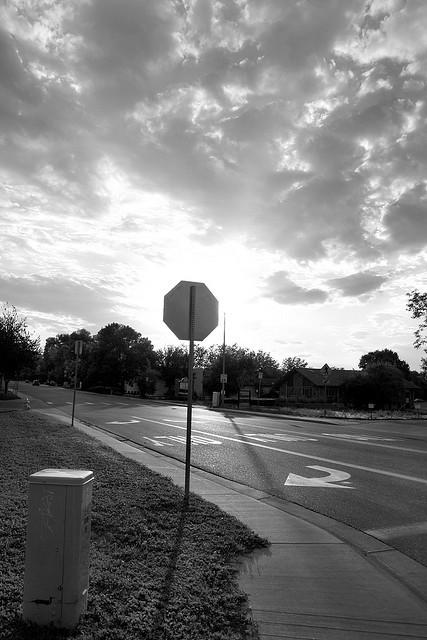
left=32, top=380, right=40, bottom=386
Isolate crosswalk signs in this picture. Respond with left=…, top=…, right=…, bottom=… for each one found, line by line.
left=321, top=364, right=332, bottom=378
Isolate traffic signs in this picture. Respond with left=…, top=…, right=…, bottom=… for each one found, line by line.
left=220, top=373, right=228, bottom=383
